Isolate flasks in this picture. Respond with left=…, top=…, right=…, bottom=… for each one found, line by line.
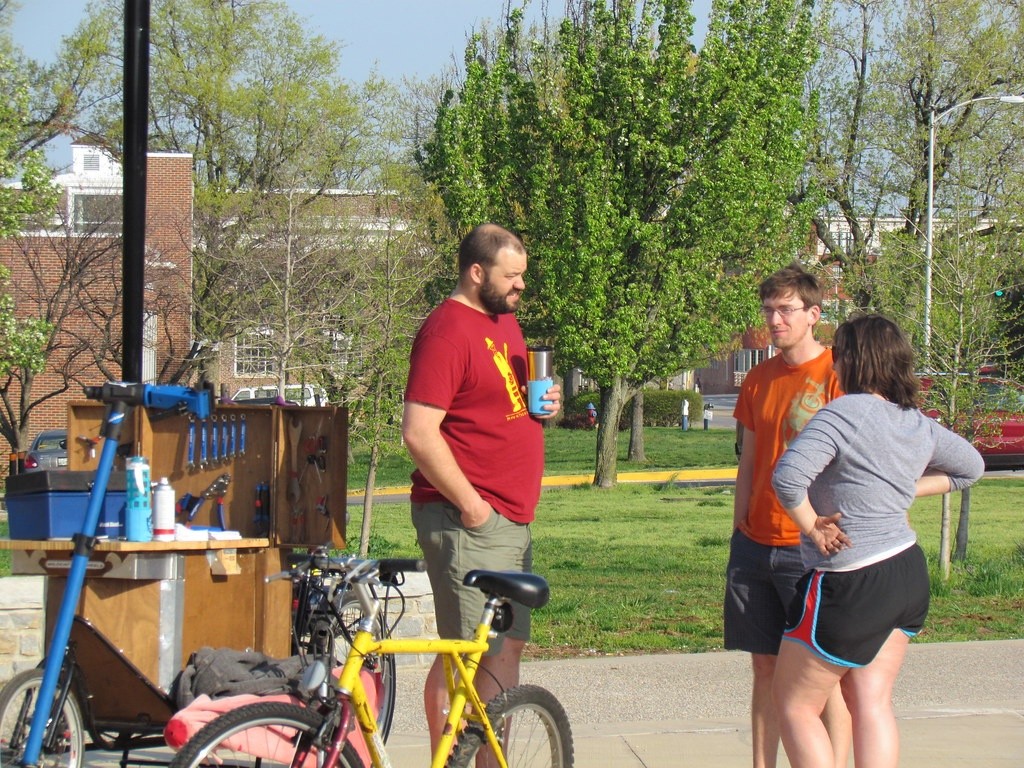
left=526, top=346, right=554, bottom=414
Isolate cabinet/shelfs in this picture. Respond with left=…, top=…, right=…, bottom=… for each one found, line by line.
left=0, top=399, right=349, bottom=734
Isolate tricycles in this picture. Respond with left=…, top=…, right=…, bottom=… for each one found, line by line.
left=0, top=381, right=399, bottom=768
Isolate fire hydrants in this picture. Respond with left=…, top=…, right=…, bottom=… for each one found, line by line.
left=585, top=401, right=597, bottom=430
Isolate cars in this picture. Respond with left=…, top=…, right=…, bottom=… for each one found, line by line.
left=23, top=429, right=68, bottom=473
left=916, top=373, right=1024, bottom=466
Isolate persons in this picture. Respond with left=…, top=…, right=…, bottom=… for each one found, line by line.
left=403, top=224, right=561, bottom=768
left=724, top=261, right=888, bottom=768
left=770, top=315, right=985, bottom=768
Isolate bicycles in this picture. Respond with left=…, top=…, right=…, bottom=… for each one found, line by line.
left=165, top=542, right=578, bottom=768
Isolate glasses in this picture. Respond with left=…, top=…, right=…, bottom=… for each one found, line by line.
left=764, top=305, right=811, bottom=317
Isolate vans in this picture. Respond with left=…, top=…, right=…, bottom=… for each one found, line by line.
left=231, top=383, right=329, bottom=407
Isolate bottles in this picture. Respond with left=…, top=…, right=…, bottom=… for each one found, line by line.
left=125, top=457, right=153, bottom=541
left=153, top=477, right=176, bottom=541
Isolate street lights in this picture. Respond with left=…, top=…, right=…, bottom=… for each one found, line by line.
left=919, top=94, right=1024, bottom=369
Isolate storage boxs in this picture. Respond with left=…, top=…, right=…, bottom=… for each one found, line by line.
left=3, top=491, right=127, bottom=541
